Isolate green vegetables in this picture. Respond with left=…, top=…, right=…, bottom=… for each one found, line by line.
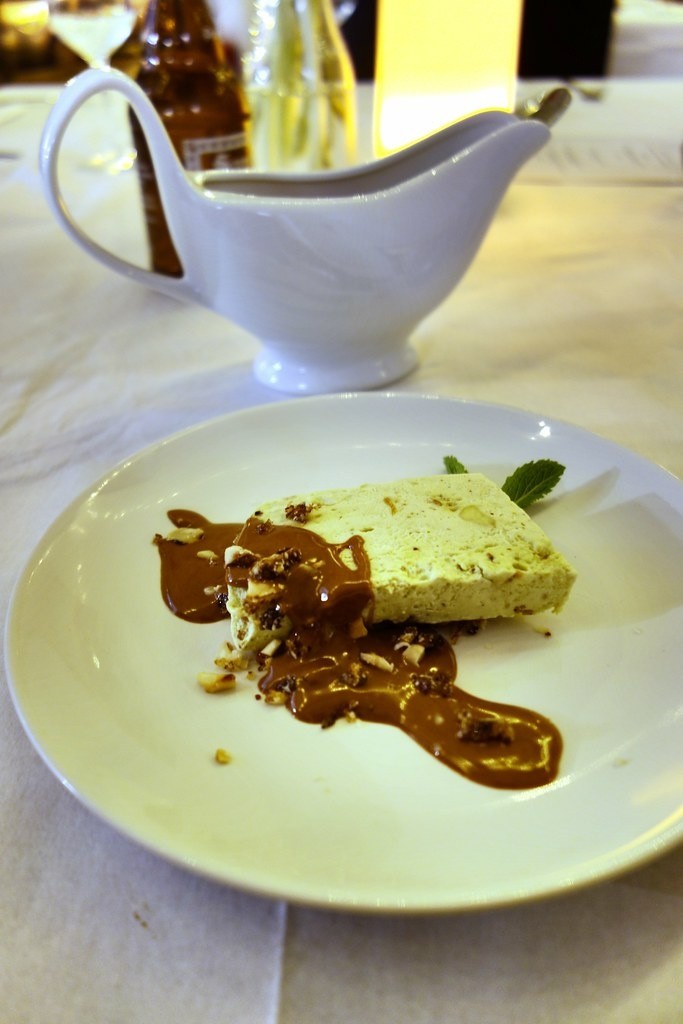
left=443, top=454, right=567, bottom=508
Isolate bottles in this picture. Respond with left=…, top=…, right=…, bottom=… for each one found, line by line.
left=242, top=0, right=357, bottom=173
left=129, top=1, right=252, bottom=277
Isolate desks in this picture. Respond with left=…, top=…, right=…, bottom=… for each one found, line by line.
left=1, top=77, right=682, bottom=1024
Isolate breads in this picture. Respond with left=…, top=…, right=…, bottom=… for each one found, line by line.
left=225, top=471, right=576, bottom=655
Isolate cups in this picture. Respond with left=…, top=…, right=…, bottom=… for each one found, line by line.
left=37, top=66, right=553, bottom=396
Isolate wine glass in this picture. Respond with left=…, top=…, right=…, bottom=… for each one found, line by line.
left=49, top=1, right=137, bottom=175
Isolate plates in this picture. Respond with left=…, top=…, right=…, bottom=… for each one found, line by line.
left=3, top=390, right=681, bottom=920
